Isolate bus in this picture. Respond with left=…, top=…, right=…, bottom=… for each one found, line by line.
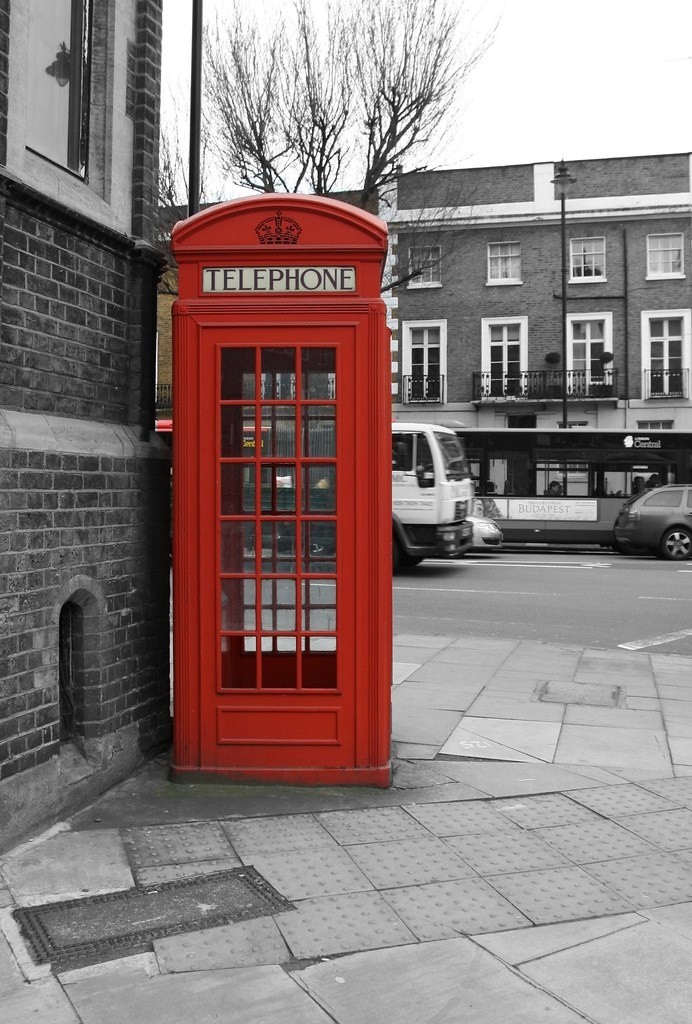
left=430, top=420, right=692, bottom=550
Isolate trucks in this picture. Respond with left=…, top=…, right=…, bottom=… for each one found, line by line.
left=154, top=416, right=503, bottom=575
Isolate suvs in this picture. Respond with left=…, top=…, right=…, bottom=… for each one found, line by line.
left=611, top=484, right=692, bottom=560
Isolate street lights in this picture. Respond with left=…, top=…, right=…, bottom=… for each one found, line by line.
left=551, top=158, right=577, bottom=499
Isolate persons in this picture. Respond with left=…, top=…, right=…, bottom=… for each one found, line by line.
left=550, top=480, right=562, bottom=494
left=646, top=473, right=660, bottom=487
